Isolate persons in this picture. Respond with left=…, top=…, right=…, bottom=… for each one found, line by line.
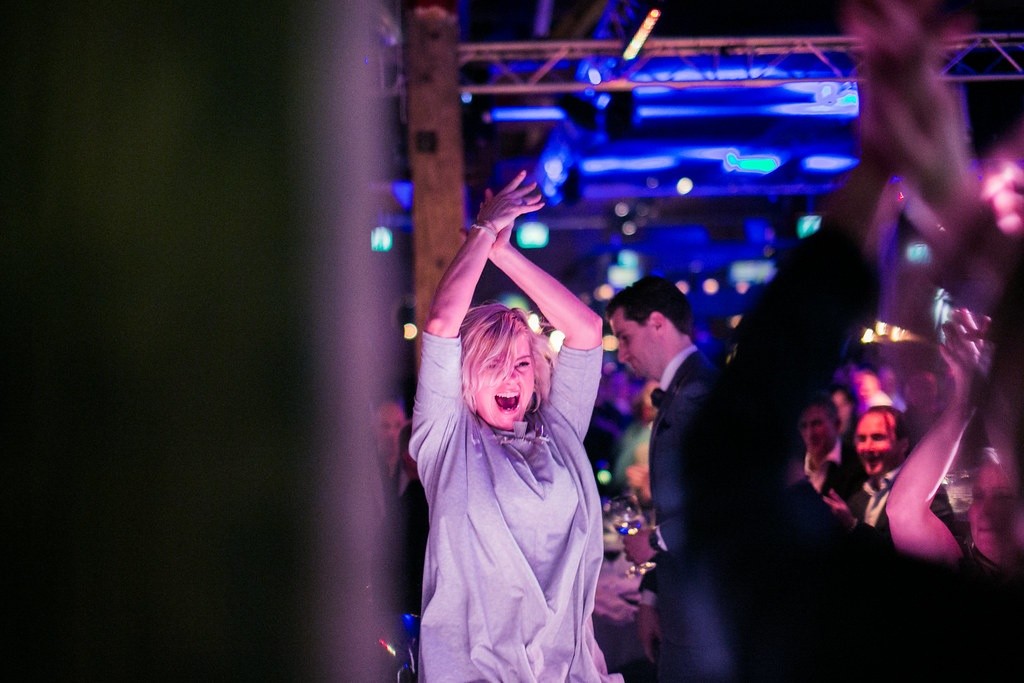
left=407, top=171, right=624, bottom=683
left=376, top=0, right=1024, bottom=683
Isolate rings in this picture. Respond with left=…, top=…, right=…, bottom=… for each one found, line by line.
left=520, top=197, right=526, bottom=207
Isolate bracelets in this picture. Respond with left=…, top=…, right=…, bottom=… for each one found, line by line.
left=470, top=224, right=496, bottom=242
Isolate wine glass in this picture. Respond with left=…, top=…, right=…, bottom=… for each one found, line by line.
left=609, top=494, right=656, bottom=579
